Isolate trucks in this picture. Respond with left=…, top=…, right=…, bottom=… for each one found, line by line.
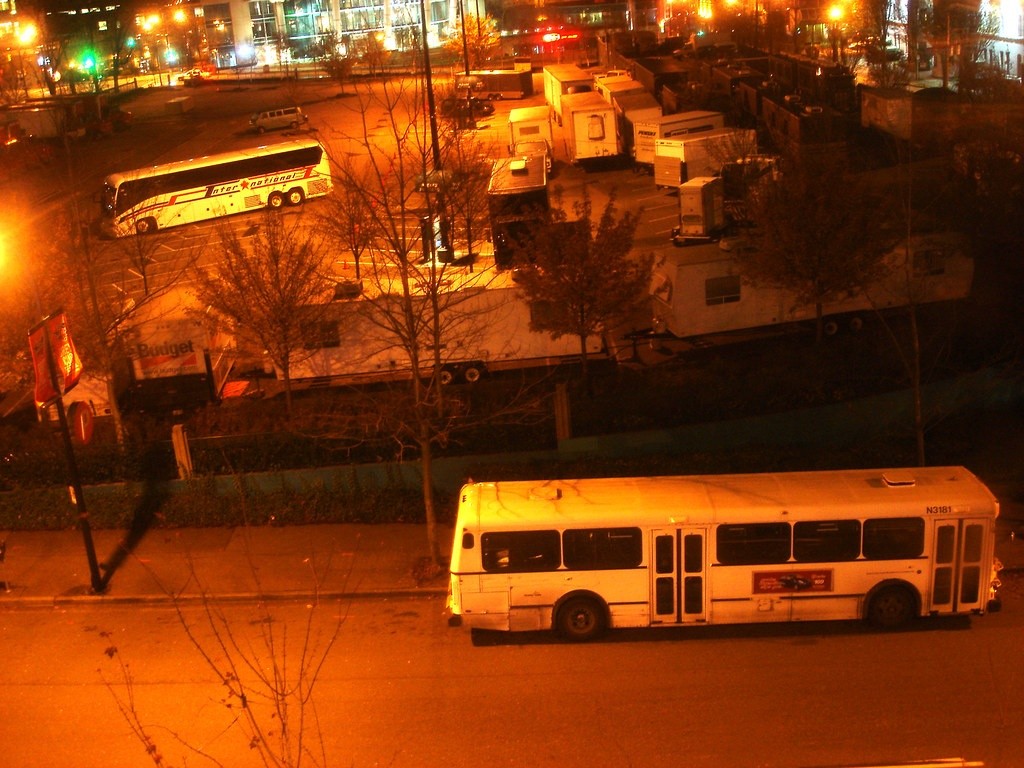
left=0, top=88, right=134, bottom=153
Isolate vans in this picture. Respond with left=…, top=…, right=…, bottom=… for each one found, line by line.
left=254, top=106, right=308, bottom=135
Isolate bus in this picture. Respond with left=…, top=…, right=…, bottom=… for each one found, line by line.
left=454, top=69, right=533, bottom=102
left=444, top=465, right=1001, bottom=644
left=91, top=138, right=334, bottom=240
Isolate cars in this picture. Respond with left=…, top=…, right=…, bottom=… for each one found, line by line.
left=177, top=68, right=211, bottom=81
left=440, top=95, right=496, bottom=116
